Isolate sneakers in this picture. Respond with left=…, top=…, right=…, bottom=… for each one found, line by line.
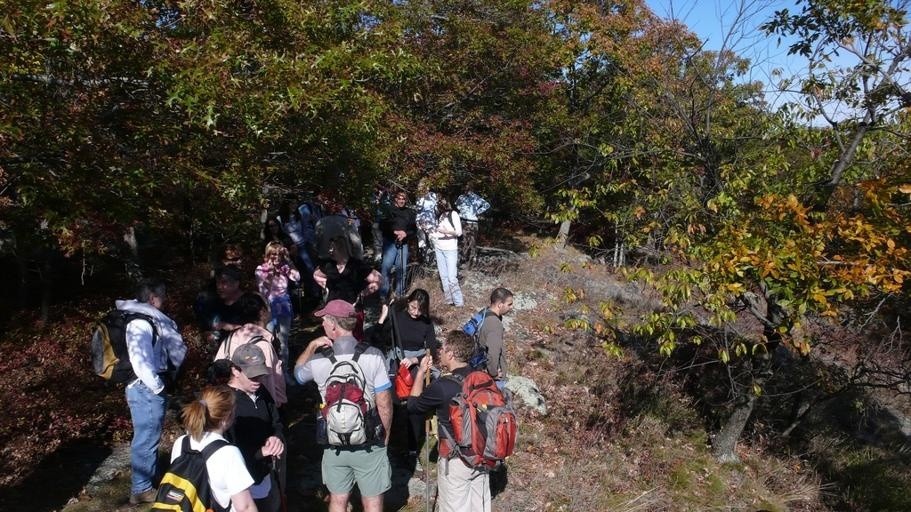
left=130, top=488, right=156, bottom=504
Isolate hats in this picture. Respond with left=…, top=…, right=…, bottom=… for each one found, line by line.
left=232, top=344, right=270, bottom=379
left=314, top=300, right=357, bottom=319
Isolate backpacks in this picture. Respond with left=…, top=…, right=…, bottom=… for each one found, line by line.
left=90, top=309, right=156, bottom=382
left=315, top=342, right=386, bottom=451
left=438, top=371, right=517, bottom=472
left=463, top=309, right=496, bottom=367
left=150, top=434, right=232, bottom=512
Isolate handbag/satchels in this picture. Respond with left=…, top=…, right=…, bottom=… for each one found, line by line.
left=395, top=361, right=423, bottom=405
left=271, top=293, right=293, bottom=319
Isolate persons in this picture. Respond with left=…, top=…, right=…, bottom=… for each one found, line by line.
left=294, top=299, right=394, bottom=510
left=154, top=175, right=515, bottom=510
left=92, top=280, right=189, bottom=506
